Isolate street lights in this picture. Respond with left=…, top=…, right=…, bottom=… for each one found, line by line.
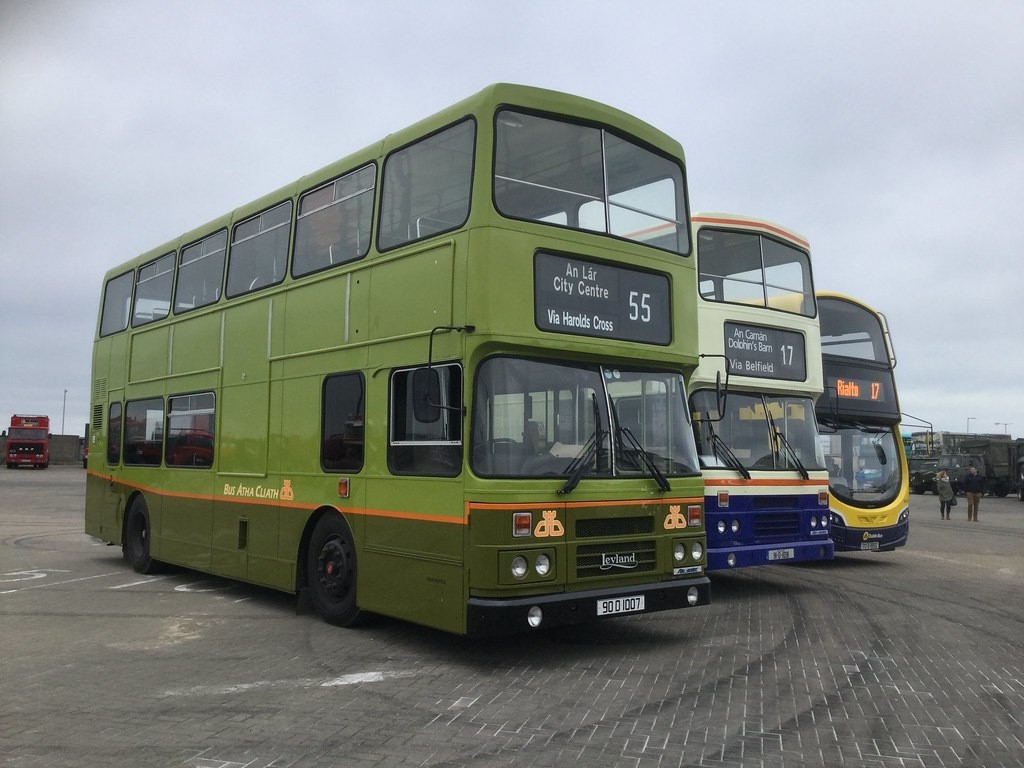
left=967, top=418, right=976, bottom=434
left=995, top=423, right=1015, bottom=435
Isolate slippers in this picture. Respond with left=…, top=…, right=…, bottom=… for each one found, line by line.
left=946, top=513, right=950, bottom=519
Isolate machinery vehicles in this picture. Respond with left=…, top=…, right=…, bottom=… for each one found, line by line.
left=908, top=430, right=1024, bottom=501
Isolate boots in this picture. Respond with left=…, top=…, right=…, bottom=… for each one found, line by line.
left=941, top=513, right=945, bottom=520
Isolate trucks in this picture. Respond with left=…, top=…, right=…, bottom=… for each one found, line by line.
left=2, top=413, right=52, bottom=469
left=109, top=416, right=155, bottom=462
left=80, top=422, right=89, bottom=469
left=173, top=430, right=214, bottom=467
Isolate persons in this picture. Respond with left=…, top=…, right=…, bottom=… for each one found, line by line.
left=937, top=472, right=952, bottom=520
left=964, top=467, right=983, bottom=522
left=855, top=466, right=866, bottom=489
left=831, top=459, right=839, bottom=477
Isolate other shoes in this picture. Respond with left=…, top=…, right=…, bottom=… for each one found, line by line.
left=974, top=517, right=978, bottom=522
left=967, top=517, right=971, bottom=521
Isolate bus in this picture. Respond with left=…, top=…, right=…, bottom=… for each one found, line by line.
left=691, top=288, right=940, bottom=555
left=82, top=75, right=727, bottom=640
left=855, top=431, right=938, bottom=493
left=488, top=210, right=837, bottom=569
